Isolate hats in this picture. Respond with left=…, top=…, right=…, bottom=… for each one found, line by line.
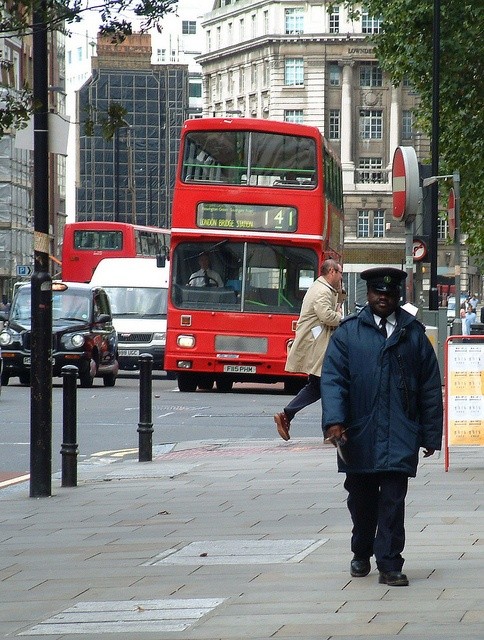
left=360, top=267, right=408, bottom=296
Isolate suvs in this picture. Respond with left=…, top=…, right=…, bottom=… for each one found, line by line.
left=0, top=279, right=119, bottom=387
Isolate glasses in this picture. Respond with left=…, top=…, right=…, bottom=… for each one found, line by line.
left=334, top=269, right=344, bottom=274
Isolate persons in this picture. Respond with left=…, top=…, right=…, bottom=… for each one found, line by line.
left=273, top=259, right=347, bottom=442
left=465, top=307, right=477, bottom=324
left=461, top=312, right=471, bottom=336
left=185, top=254, right=224, bottom=288
left=319, top=268, right=443, bottom=586
left=469, top=295, right=479, bottom=309
left=461, top=299, right=473, bottom=310
left=282, top=173, right=300, bottom=185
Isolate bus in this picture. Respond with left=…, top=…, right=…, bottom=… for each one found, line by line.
left=162, top=117, right=345, bottom=401
left=59, top=221, right=169, bottom=284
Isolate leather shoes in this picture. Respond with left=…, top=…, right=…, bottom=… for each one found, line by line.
left=378, top=571, right=409, bottom=586
left=350, top=554, right=371, bottom=579
left=274, top=412, right=293, bottom=442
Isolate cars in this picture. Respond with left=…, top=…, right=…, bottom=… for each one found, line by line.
left=446, top=296, right=467, bottom=328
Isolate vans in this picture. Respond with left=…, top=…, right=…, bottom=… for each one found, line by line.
left=88, top=257, right=172, bottom=371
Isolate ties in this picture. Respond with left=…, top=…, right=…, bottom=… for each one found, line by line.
left=379, top=318, right=387, bottom=337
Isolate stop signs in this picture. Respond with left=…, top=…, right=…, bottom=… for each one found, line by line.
left=447, top=187, right=456, bottom=241
left=388, top=146, right=421, bottom=223
